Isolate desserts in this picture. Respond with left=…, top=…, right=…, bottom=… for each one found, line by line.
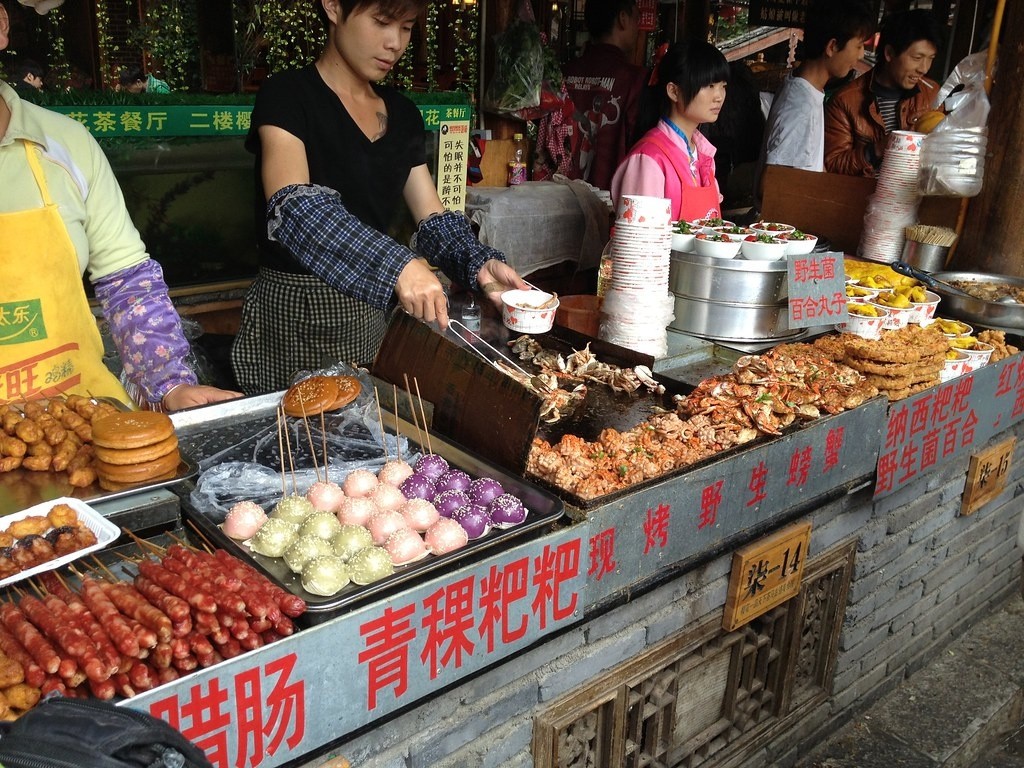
left=221, top=453, right=528, bottom=596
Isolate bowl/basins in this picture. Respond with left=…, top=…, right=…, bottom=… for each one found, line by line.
left=834, top=275, right=995, bottom=383
left=599, top=194, right=672, bottom=359
left=858, top=130, right=929, bottom=264
left=501, top=289, right=560, bottom=334
left=919, top=271, right=1024, bottom=330
left=670, top=218, right=818, bottom=262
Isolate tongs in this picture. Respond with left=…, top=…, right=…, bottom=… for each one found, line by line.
left=400, top=291, right=554, bottom=397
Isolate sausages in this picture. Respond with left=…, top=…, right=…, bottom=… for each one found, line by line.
left=0, top=545, right=307, bottom=707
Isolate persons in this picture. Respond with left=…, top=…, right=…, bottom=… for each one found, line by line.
left=235, top=1, right=534, bottom=400
left=13, top=60, right=46, bottom=102
left=523, top=1, right=649, bottom=189
left=1, top=6, right=251, bottom=411
left=609, top=38, right=730, bottom=235
left=117, top=65, right=172, bottom=95
left=750, top=0, right=948, bottom=205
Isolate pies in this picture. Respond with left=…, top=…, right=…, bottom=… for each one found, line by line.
left=770, top=326, right=950, bottom=401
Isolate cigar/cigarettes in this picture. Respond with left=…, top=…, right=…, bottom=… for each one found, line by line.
left=918, top=77, right=933, bottom=90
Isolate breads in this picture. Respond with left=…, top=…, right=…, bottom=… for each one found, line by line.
left=330, top=375, right=363, bottom=411
left=280, top=374, right=338, bottom=417
left=91, top=410, right=180, bottom=492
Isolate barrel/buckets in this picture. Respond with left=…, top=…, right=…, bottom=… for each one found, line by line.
left=556, top=294, right=602, bottom=337
left=900, top=238, right=950, bottom=274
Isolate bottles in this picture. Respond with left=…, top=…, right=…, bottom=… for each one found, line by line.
left=461, top=291, right=482, bottom=343
left=596, top=227, right=616, bottom=298
left=506, top=133, right=527, bottom=185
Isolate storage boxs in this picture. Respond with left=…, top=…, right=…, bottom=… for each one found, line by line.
left=471, top=138, right=529, bottom=188
left=81, top=129, right=438, bottom=302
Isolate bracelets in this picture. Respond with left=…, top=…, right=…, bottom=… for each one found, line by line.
left=162, top=382, right=185, bottom=413
